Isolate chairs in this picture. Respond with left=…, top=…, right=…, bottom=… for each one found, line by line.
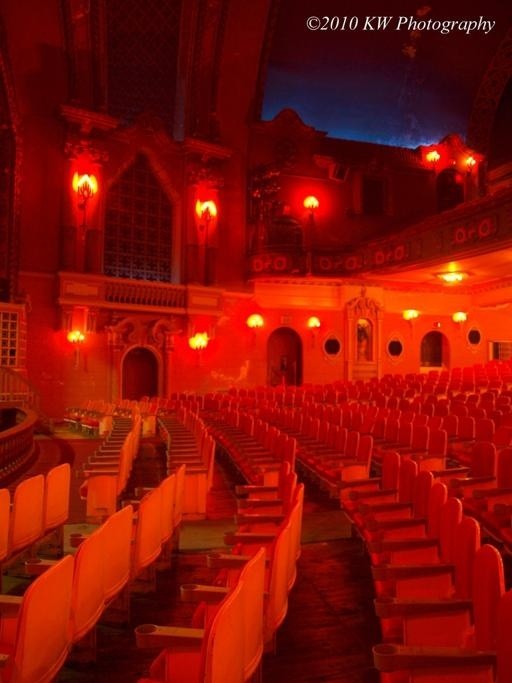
left=0, top=357, right=512, bottom=683
